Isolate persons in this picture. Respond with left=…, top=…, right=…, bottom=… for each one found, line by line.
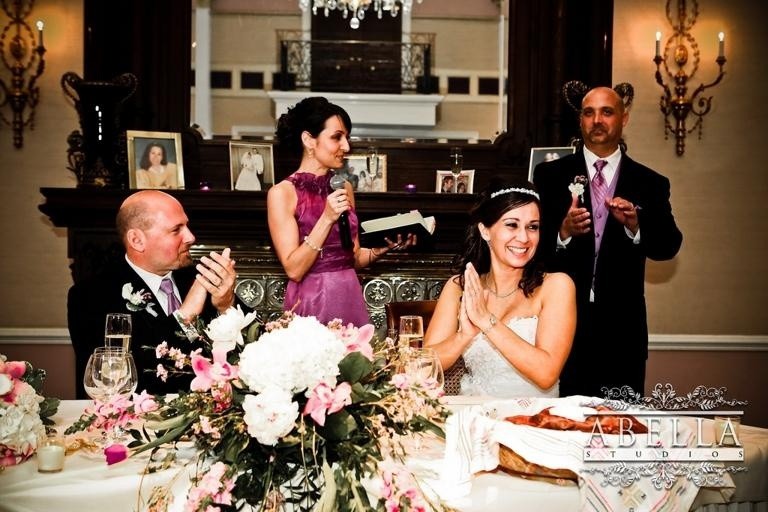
left=534, top=87, right=683, bottom=398
left=136, top=142, right=177, bottom=190
left=67, top=190, right=239, bottom=398
left=235, top=152, right=261, bottom=190
left=457, top=182, right=465, bottom=194
left=421, top=180, right=577, bottom=398
left=251, top=149, right=264, bottom=189
left=267, top=96, right=438, bottom=327
left=441, top=176, right=454, bottom=193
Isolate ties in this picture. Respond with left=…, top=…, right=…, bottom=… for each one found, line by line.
left=159, top=279, right=181, bottom=315
left=590, top=159, right=609, bottom=206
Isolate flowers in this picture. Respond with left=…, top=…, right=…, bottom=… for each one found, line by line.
left=63, top=299, right=456, bottom=512
left=0, top=354, right=53, bottom=471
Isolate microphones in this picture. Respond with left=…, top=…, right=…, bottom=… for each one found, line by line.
left=329, top=174, right=354, bottom=253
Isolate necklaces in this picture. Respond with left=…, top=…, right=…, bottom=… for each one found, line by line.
left=484, top=271, right=524, bottom=298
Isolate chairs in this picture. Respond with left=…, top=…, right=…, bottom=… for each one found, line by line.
left=384, top=304, right=446, bottom=391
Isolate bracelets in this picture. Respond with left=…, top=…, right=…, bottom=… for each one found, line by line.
left=304, top=237, right=323, bottom=253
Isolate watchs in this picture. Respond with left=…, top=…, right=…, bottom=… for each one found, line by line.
left=481, top=311, right=498, bottom=335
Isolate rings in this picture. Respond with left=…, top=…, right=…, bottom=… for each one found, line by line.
left=217, top=279, right=224, bottom=288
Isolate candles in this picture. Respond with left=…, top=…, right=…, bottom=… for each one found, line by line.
left=34, top=20, right=47, bottom=47
left=654, top=31, right=665, bottom=57
left=718, top=32, right=727, bottom=61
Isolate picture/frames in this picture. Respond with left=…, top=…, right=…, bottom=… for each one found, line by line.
left=126, top=130, right=186, bottom=190
left=436, top=169, right=474, bottom=195
left=331, top=153, right=387, bottom=195
left=228, top=141, right=274, bottom=190
left=529, top=146, right=575, bottom=182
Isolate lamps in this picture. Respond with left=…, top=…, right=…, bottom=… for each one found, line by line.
left=299, top=0, right=425, bottom=27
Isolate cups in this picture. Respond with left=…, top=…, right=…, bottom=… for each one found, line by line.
left=104, top=311, right=132, bottom=353
left=35, top=435, right=68, bottom=475
left=364, top=146, right=380, bottom=193
left=83, top=346, right=139, bottom=402
left=449, top=145, right=463, bottom=194
left=399, top=313, right=424, bottom=355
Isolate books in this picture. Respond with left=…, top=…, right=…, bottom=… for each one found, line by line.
left=361, top=210, right=435, bottom=248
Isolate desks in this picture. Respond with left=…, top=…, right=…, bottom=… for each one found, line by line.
left=35, top=185, right=520, bottom=399
